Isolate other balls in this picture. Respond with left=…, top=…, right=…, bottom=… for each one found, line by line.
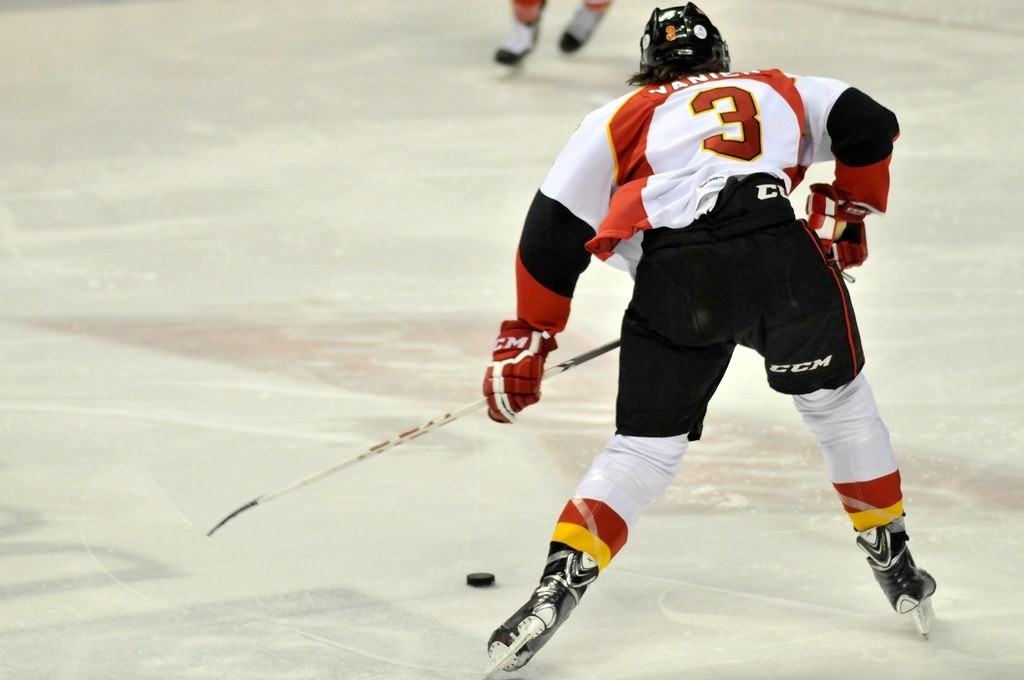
left=465, top=572, right=495, bottom=587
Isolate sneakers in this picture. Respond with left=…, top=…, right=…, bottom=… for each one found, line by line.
left=560, top=6, right=602, bottom=52
left=856, top=513, right=937, bottom=640
left=485, top=541, right=599, bottom=680
left=493, top=22, right=537, bottom=74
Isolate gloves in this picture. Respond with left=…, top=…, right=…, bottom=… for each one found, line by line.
left=804, top=192, right=870, bottom=270
left=483, top=320, right=558, bottom=423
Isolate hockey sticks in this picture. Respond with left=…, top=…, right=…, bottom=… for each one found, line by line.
left=208, top=337, right=620, bottom=537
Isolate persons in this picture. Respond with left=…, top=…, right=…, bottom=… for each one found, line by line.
left=497, top=0, right=612, bottom=63
left=485, top=2, right=935, bottom=680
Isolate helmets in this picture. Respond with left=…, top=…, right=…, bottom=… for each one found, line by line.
left=639, top=1, right=729, bottom=76
